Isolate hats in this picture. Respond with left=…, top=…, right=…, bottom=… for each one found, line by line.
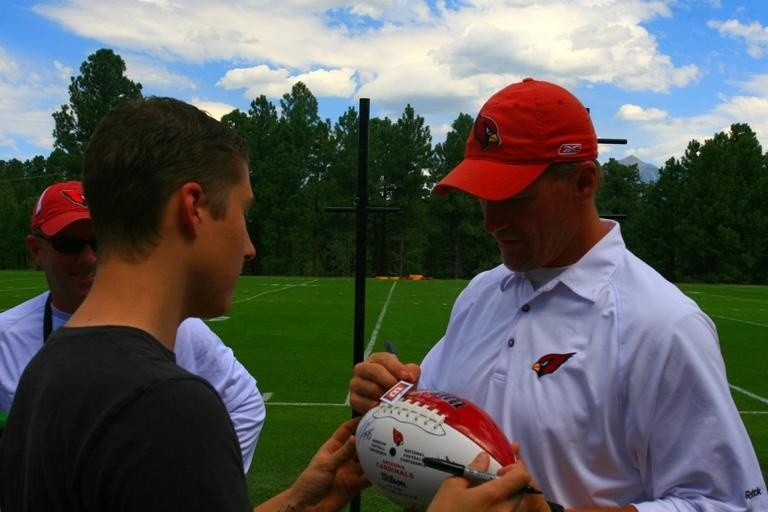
left=431, top=79, right=597, bottom=203
left=31, top=181, right=94, bottom=237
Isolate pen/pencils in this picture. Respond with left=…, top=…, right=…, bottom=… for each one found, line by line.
left=423, top=457, right=543, bottom=495
left=385, top=341, right=393, bottom=353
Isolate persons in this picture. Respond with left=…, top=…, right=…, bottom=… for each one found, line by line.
left=1, top=181, right=266, bottom=474
left=349, top=79, right=768, bottom=512
left=2, top=96, right=537, bottom=511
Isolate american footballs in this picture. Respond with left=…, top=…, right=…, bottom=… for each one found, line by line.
left=356, top=390, right=517, bottom=511
